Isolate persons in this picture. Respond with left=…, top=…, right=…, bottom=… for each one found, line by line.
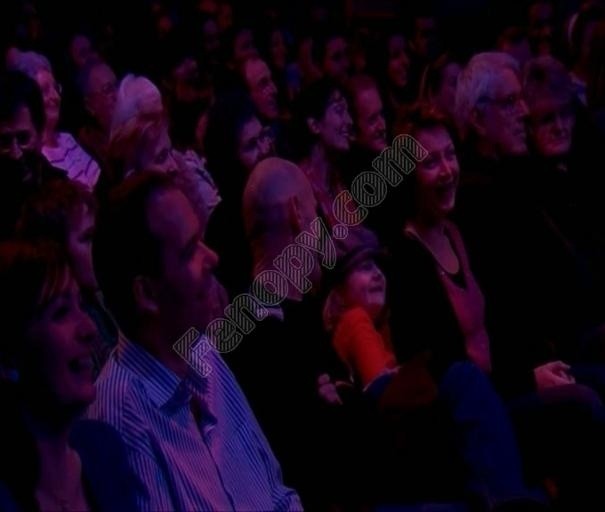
left=0, top=1, right=605, bottom=511
